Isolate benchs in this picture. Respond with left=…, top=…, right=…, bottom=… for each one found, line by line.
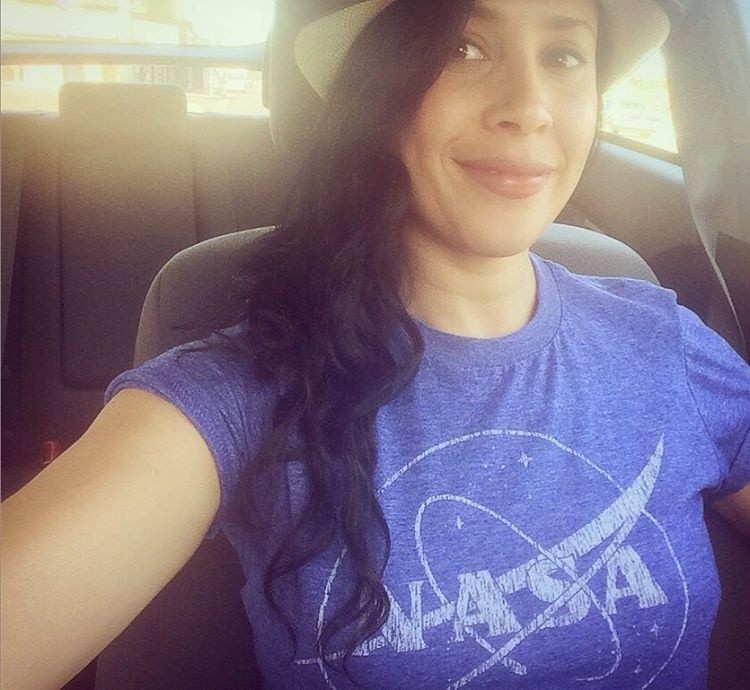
left=0, top=84, right=271, bottom=495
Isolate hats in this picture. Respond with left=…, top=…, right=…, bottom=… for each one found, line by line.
left=294, top=2, right=670, bottom=108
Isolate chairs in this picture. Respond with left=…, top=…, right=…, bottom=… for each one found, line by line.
left=90, top=4, right=668, bottom=689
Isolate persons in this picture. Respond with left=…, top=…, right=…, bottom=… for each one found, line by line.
left=0, top=1, right=750, bottom=689
